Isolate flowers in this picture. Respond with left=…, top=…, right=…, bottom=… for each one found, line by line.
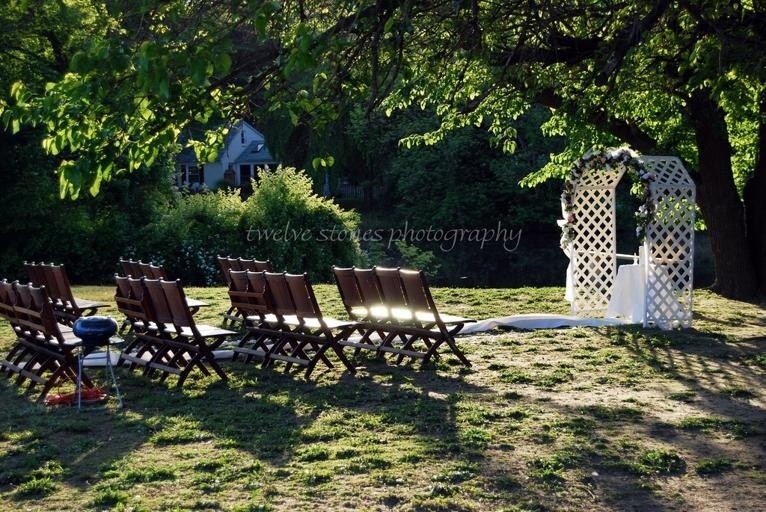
left=559, top=144, right=658, bottom=249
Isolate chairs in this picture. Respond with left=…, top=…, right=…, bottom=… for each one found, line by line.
left=0, top=253, right=475, bottom=405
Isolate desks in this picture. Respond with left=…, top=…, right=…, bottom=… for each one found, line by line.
left=614, top=264, right=673, bottom=321
